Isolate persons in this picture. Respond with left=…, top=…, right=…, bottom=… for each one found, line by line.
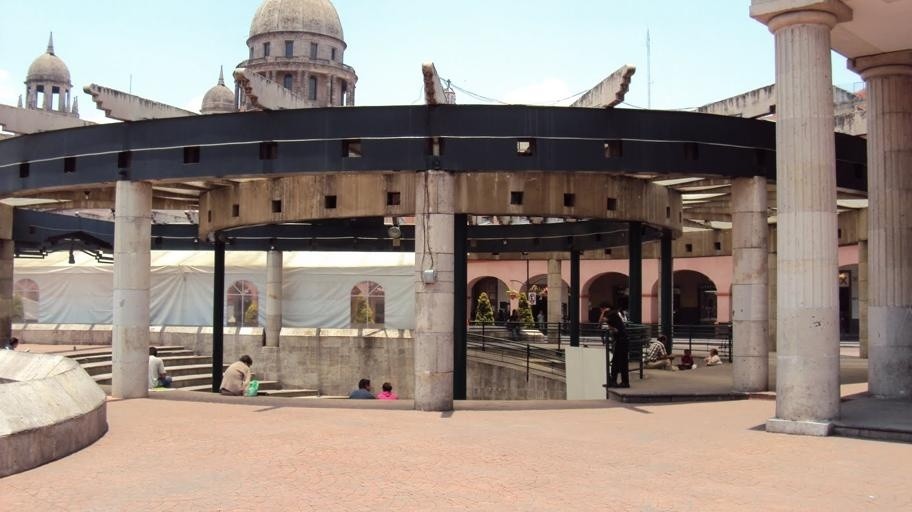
left=598, top=302, right=630, bottom=387
left=376, top=382, right=399, bottom=400
left=4, top=338, right=18, bottom=350
left=644, top=335, right=675, bottom=371
left=220, top=355, right=252, bottom=395
left=703, top=349, right=721, bottom=366
left=679, top=350, right=694, bottom=369
left=495, top=303, right=627, bottom=346
left=149, top=347, right=173, bottom=388
left=349, top=379, right=375, bottom=398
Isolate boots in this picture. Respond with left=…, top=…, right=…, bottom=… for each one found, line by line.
left=617, top=376, right=632, bottom=387
left=602, top=375, right=617, bottom=388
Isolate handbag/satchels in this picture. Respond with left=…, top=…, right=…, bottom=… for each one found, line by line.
left=244, top=379, right=260, bottom=397
